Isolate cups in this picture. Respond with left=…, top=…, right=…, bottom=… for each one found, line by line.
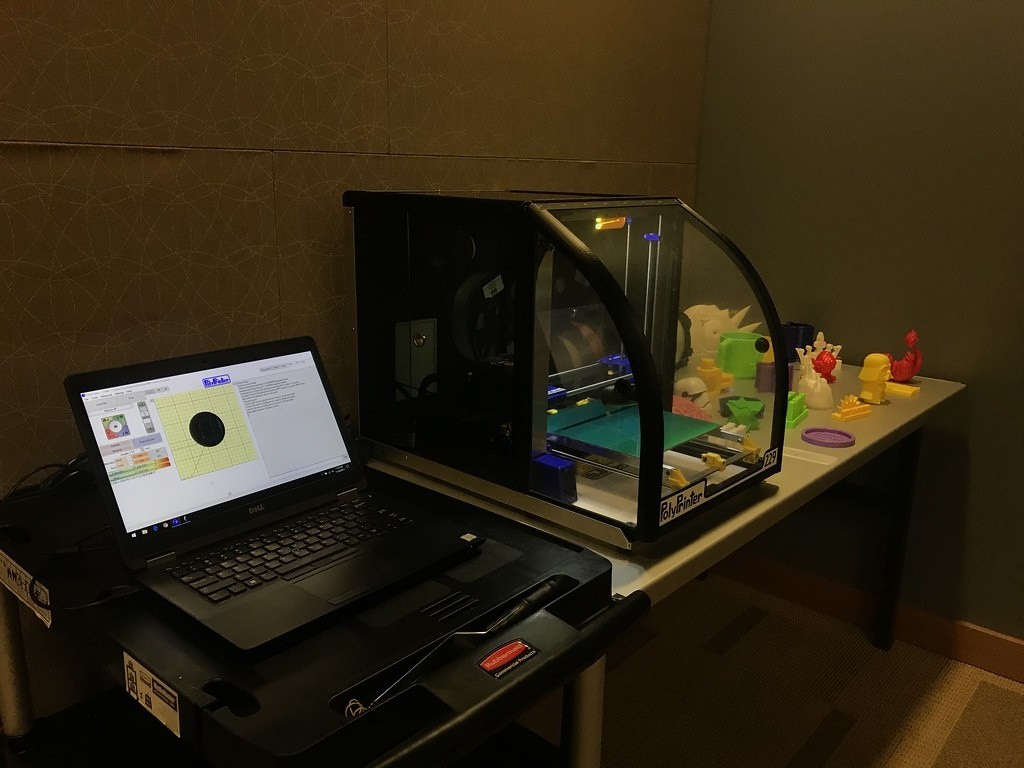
left=716, top=332, right=763, bottom=379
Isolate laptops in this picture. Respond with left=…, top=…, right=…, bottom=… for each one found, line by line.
left=64, top=335, right=488, bottom=661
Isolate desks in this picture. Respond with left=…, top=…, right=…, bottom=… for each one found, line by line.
left=1, top=360, right=970, bottom=768
